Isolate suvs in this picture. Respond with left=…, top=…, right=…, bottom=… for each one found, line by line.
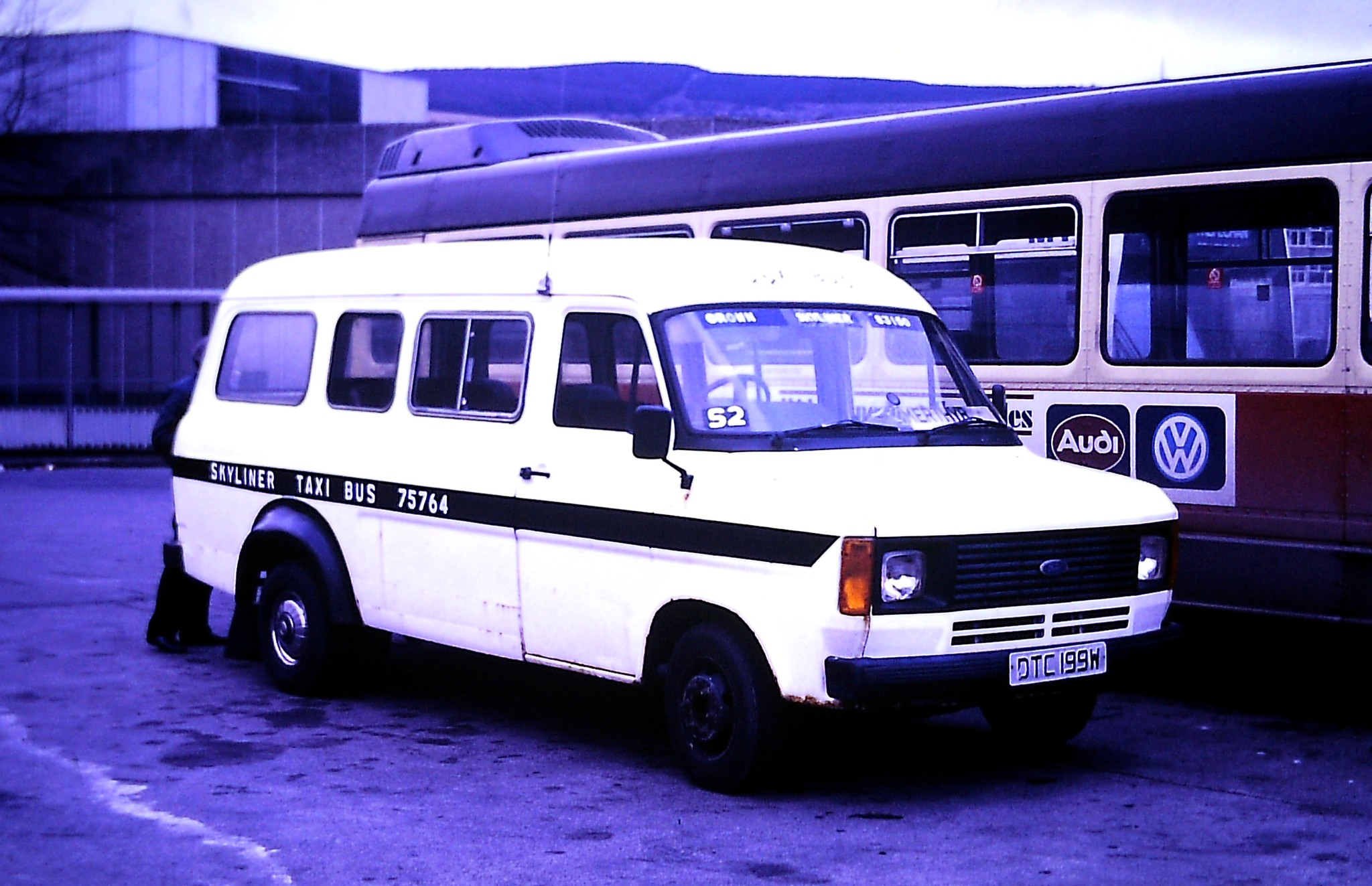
left=172, top=240, right=1178, bottom=795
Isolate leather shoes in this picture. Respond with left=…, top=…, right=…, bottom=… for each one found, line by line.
left=147, top=633, right=187, bottom=654
left=180, top=630, right=228, bottom=645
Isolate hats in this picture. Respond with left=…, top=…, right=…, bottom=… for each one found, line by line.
left=194, top=336, right=208, bottom=362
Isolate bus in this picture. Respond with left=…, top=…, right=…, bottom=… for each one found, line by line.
left=344, top=56, right=1372, bottom=632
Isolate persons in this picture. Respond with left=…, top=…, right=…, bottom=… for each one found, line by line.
left=145, top=337, right=229, bottom=654
